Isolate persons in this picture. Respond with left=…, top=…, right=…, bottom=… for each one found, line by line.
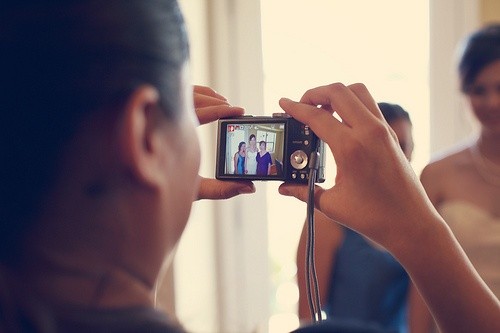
left=234, top=134, right=272, bottom=174
left=296, top=25, right=500, bottom=333
left=0, top=0, right=500, bottom=333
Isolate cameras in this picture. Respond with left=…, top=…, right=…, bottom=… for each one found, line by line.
left=216, top=113, right=325, bottom=185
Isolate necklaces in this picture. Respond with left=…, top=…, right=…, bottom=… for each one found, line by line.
left=467, top=149, right=500, bottom=189
